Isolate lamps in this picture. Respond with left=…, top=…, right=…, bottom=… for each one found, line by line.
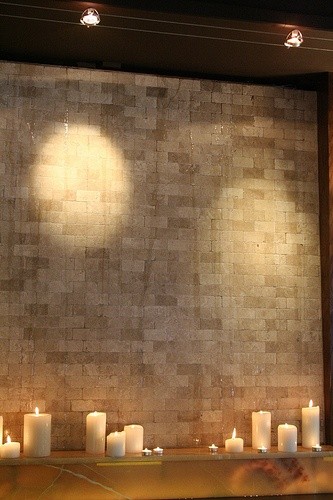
left=284, top=30, right=303, bottom=48
left=80, top=8, right=100, bottom=27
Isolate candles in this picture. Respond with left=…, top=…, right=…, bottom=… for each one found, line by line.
left=302, top=399, right=321, bottom=448
left=0, top=435, right=20, bottom=459
left=225, top=427, right=245, bottom=453
left=24, top=407, right=53, bottom=457
left=86, top=410, right=107, bottom=455
left=107, top=431, right=127, bottom=457
left=312, top=444, right=322, bottom=452
left=258, top=446, right=268, bottom=453
left=153, top=446, right=164, bottom=456
left=278, top=423, right=299, bottom=452
left=209, top=443, right=219, bottom=453
left=142, top=448, right=153, bottom=456
left=124, top=423, right=144, bottom=453
left=252, top=409, right=273, bottom=450
